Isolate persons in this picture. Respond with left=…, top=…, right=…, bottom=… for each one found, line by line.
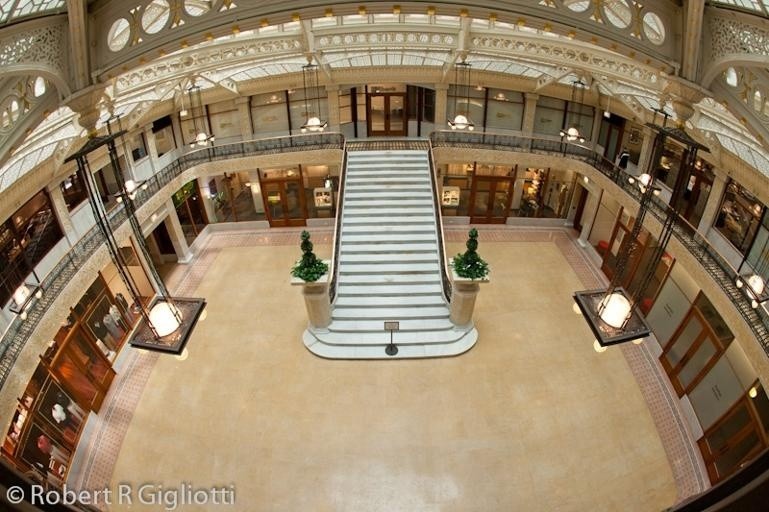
left=31, top=462, right=63, bottom=487
left=56, top=392, right=87, bottom=422
left=37, top=435, right=69, bottom=466
left=50, top=403, right=79, bottom=433
left=617, top=147, right=630, bottom=173
left=94, top=293, right=135, bottom=351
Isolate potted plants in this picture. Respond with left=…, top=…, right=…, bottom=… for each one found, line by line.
left=446, top=226, right=492, bottom=326
left=288, top=229, right=332, bottom=329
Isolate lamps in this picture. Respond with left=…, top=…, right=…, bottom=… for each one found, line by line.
left=735, top=234, right=769, bottom=309
left=298, top=62, right=328, bottom=133
left=63, top=117, right=208, bottom=362
left=570, top=105, right=717, bottom=354
left=559, top=79, right=586, bottom=143
left=447, top=56, right=475, bottom=131
left=187, top=83, right=215, bottom=151
